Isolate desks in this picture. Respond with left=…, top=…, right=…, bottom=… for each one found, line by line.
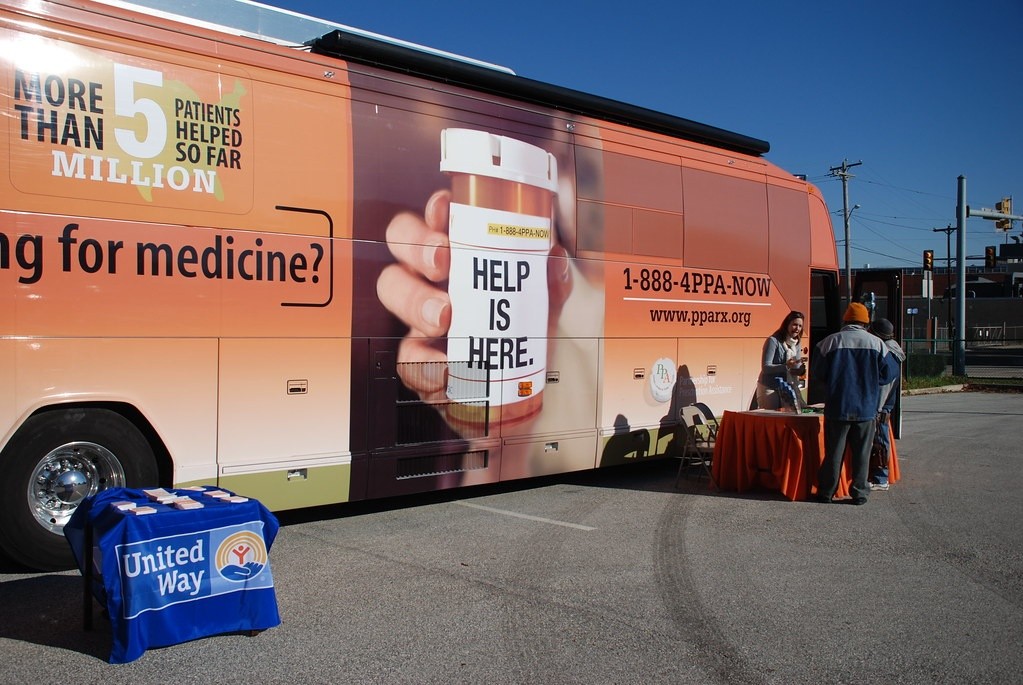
left=58, top=486, right=281, bottom=665
left=710, top=409, right=900, bottom=499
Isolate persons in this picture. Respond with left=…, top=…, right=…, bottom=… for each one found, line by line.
left=376, top=176, right=606, bottom=488
left=868, top=319, right=907, bottom=491
left=756, top=311, right=809, bottom=409
left=814, top=302, right=900, bottom=505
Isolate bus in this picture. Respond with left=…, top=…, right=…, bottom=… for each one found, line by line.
left=1, top=2, right=867, bottom=573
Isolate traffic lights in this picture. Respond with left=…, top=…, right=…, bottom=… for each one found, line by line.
left=923, top=250, right=933, bottom=274
left=996, top=197, right=1011, bottom=229
left=984, top=245, right=997, bottom=270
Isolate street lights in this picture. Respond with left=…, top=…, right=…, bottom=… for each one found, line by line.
left=844, top=205, right=861, bottom=308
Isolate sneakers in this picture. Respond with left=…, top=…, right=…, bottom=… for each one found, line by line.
left=868, top=481, right=888, bottom=491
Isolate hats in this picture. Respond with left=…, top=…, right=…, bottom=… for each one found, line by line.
left=870, top=317, right=894, bottom=339
left=843, top=302, right=870, bottom=323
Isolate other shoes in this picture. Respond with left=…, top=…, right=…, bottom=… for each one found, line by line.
left=852, top=497, right=868, bottom=505
left=814, top=496, right=832, bottom=503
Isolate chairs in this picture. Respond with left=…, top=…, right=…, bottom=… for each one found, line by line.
left=672, top=402, right=721, bottom=493
